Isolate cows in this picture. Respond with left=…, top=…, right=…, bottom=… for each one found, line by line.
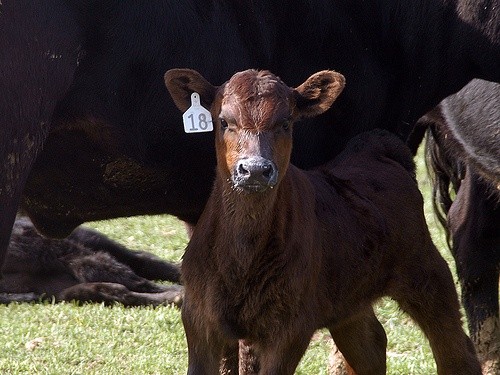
left=0, top=218, right=183, bottom=307
left=0, top=0, right=499, bottom=281
left=165, top=68, right=482, bottom=375
left=407, top=77, right=499, bottom=375
